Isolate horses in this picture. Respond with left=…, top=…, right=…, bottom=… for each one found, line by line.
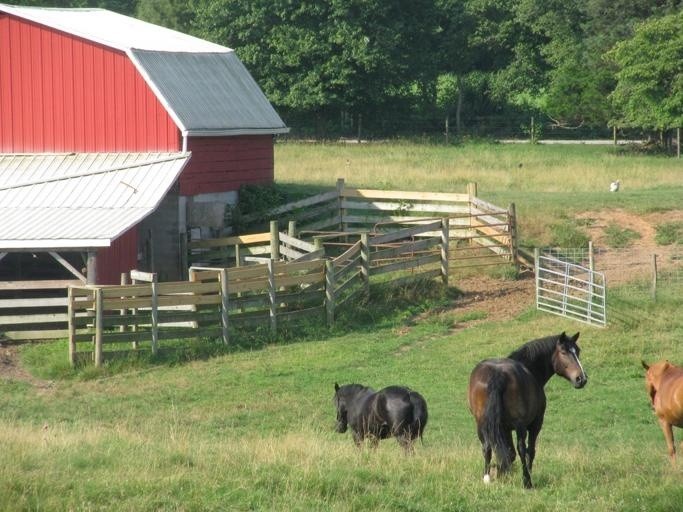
left=466, top=331, right=589, bottom=490
left=640, top=359, right=683, bottom=470
left=331, top=382, right=428, bottom=456
